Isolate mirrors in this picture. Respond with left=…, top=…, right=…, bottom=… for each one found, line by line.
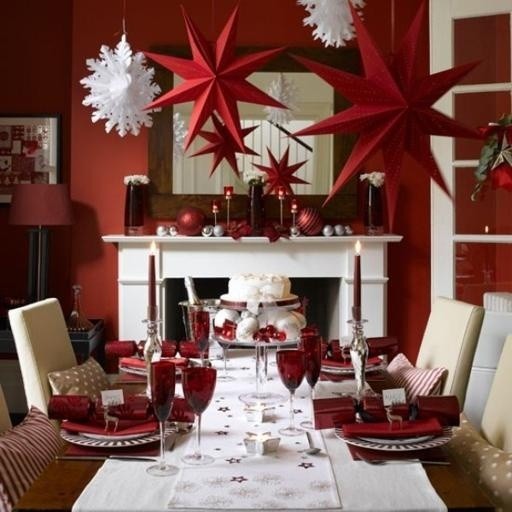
left=171, top=71, right=336, bottom=196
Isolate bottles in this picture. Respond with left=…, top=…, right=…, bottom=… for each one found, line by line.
left=182, top=274, right=203, bottom=306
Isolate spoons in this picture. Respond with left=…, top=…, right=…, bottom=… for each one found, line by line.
left=303, top=430, right=322, bottom=456
left=354, top=451, right=452, bottom=467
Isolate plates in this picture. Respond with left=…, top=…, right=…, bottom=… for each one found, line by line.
left=321, top=364, right=375, bottom=370
left=78, top=429, right=152, bottom=441
left=117, top=360, right=202, bottom=376
left=59, top=413, right=181, bottom=449
left=127, top=364, right=179, bottom=373
left=355, top=435, right=436, bottom=445
left=320, top=359, right=388, bottom=375
left=332, top=422, right=455, bottom=452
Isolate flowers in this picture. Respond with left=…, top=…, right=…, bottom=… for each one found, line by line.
left=123, top=174, right=152, bottom=185
left=243, top=169, right=263, bottom=183
left=360, top=171, right=385, bottom=187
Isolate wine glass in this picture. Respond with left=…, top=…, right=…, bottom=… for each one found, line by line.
left=275, top=348, right=307, bottom=436
left=189, top=311, right=210, bottom=367
left=144, top=360, right=182, bottom=478
left=182, top=366, right=218, bottom=465
left=212, top=317, right=238, bottom=382
left=298, top=333, right=322, bottom=430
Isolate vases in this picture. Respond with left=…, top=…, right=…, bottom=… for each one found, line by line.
left=364, top=183, right=384, bottom=235
left=124, top=185, right=144, bottom=237
left=251, top=184, right=264, bottom=227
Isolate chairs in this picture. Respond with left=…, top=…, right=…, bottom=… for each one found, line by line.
left=1, top=289, right=512, bottom=512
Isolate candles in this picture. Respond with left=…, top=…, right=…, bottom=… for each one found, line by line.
left=149, top=255, right=158, bottom=321
left=353, top=255, right=362, bottom=320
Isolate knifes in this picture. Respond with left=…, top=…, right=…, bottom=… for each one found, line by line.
left=54, top=453, right=158, bottom=464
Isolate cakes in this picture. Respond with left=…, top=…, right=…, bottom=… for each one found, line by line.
left=214, top=271, right=307, bottom=343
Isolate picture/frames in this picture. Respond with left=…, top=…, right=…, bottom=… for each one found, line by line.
left=0, top=113, right=66, bottom=209
